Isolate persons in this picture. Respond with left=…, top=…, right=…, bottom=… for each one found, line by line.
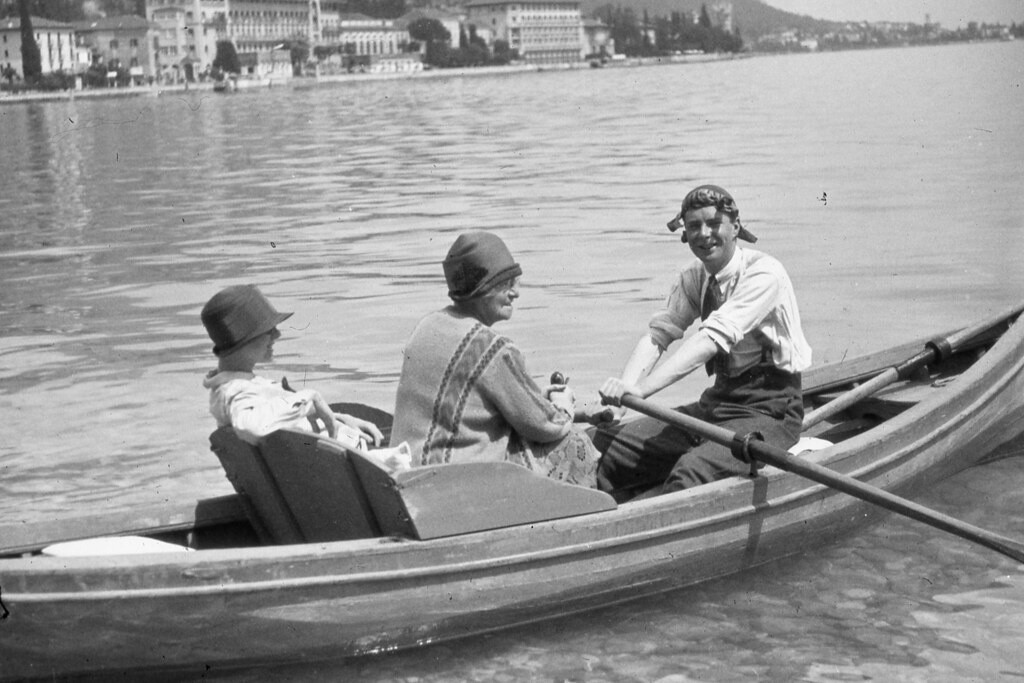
left=596, top=184, right=812, bottom=505
left=199, top=283, right=388, bottom=481
left=388, top=231, right=598, bottom=491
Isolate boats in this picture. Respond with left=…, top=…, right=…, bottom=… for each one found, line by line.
left=0, top=299, right=1024, bottom=683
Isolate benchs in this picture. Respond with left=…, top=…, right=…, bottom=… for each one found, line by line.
left=207, top=402, right=619, bottom=549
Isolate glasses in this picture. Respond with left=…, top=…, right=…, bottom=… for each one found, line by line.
left=494, top=278, right=516, bottom=292
left=265, top=326, right=277, bottom=334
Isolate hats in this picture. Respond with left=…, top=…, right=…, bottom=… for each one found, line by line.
left=681, top=185, right=759, bottom=242
left=442, top=233, right=522, bottom=302
left=200, top=285, right=296, bottom=357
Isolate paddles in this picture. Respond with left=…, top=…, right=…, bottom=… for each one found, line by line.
left=800, top=299, right=1024, bottom=433
left=621, top=392, right=1023, bottom=567
